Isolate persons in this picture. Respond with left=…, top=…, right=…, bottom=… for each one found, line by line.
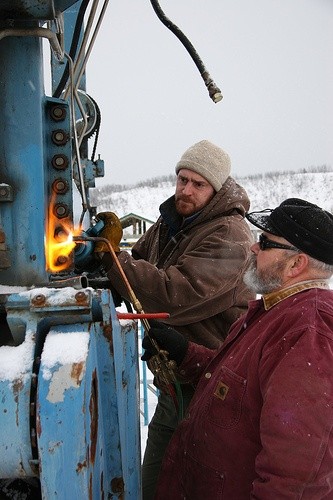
left=139, top=197, right=333, bottom=500
left=92, top=139, right=258, bottom=500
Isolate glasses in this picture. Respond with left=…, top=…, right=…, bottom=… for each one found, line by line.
left=258, top=233, right=305, bottom=255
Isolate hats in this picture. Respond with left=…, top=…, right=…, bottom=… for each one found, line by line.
left=176, top=140, right=231, bottom=193
left=246, top=198, right=333, bottom=266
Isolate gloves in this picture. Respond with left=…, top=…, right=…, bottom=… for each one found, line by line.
left=95, top=212, right=123, bottom=254
left=140, top=327, right=188, bottom=367
